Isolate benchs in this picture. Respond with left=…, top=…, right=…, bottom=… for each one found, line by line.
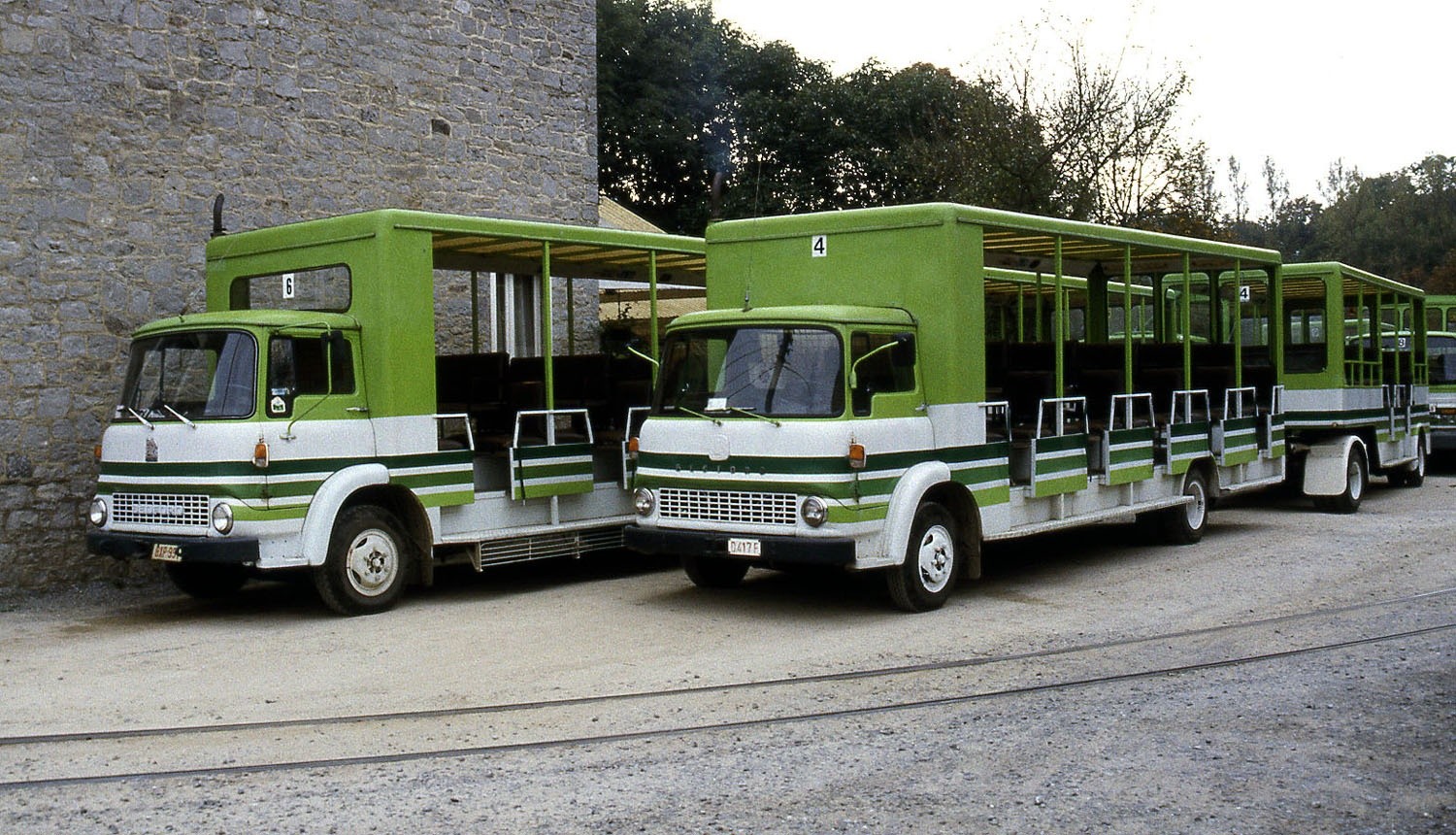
left=432, top=349, right=510, bottom=435
left=983, top=343, right=1455, bottom=423
left=608, top=357, right=653, bottom=431
left=509, top=356, right=616, bottom=438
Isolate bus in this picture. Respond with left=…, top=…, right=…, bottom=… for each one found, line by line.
left=89, top=191, right=1456, bottom=616
